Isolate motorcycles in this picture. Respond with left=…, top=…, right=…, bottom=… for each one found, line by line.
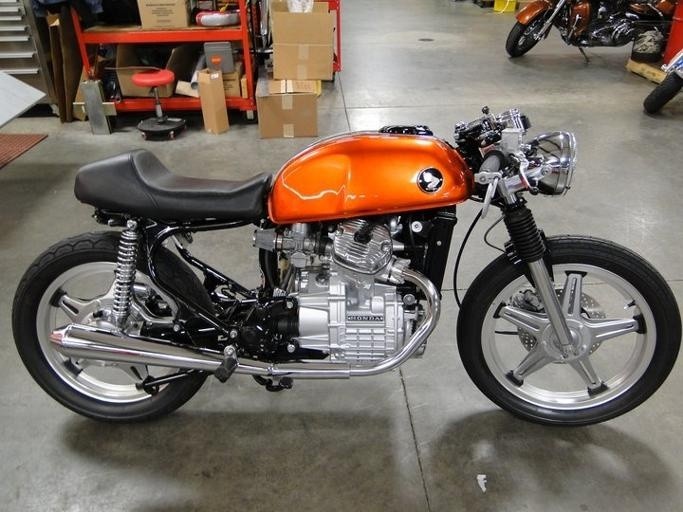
left=505, top=0, right=683, bottom=114
left=12, top=107, right=682, bottom=427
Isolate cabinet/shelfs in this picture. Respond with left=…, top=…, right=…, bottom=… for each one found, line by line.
left=68, top=0, right=257, bottom=121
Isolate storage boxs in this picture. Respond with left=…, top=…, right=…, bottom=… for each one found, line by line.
left=114, top=43, right=242, bottom=98
left=137, top=0, right=191, bottom=30
left=253, top=0, right=337, bottom=139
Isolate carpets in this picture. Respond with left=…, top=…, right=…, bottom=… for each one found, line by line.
left=0, top=133, right=49, bottom=170
